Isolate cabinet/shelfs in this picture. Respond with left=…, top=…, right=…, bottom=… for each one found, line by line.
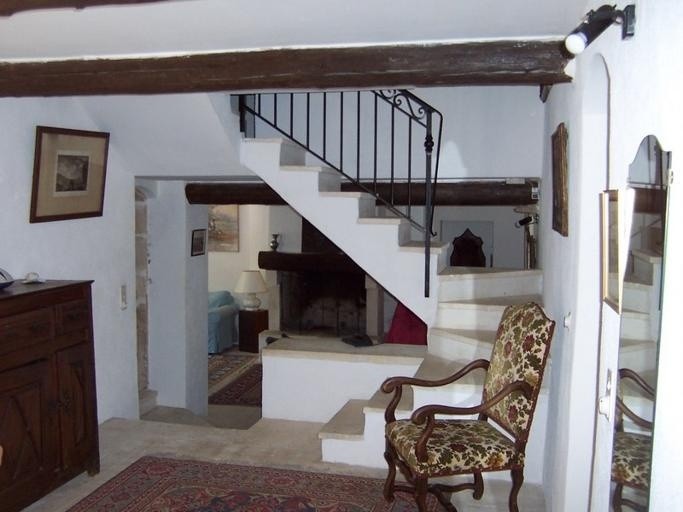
left=0, top=276, right=101, bottom=510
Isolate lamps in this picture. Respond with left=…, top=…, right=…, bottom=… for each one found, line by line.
left=234, top=269, right=267, bottom=311
left=561, top=1, right=637, bottom=53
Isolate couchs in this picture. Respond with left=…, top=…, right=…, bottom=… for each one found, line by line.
left=207, top=290, right=235, bottom=353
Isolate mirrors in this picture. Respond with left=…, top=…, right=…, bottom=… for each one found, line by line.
left=604, top=134, right=673, bottom=512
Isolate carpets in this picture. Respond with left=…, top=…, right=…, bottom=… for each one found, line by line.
left=59, top=454, right=454, bottom=511
left=208, top=354, right=261, bottom=407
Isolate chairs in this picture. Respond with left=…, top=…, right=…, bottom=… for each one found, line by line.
left=608, top=362, right=656, bottom=511
left=379, top=298, right=556, bottom=511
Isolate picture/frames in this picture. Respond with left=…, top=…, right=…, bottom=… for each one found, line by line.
left=191, top=225, right=210, bottom=256
left=207, top=204, right=239, bottom=253
left=28, top=121, right=110, bottom=222
